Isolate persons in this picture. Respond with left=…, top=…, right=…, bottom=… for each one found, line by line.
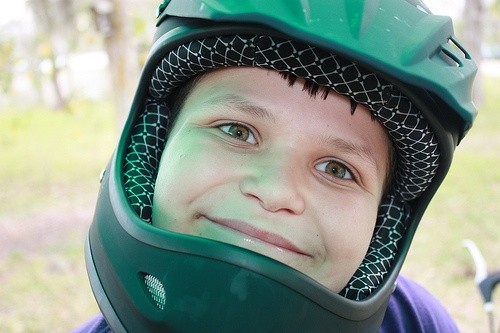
left=66, top=0, right=461, bottom=332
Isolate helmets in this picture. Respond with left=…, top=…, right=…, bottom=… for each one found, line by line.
left=84, top=0, right=478, bottom=332
